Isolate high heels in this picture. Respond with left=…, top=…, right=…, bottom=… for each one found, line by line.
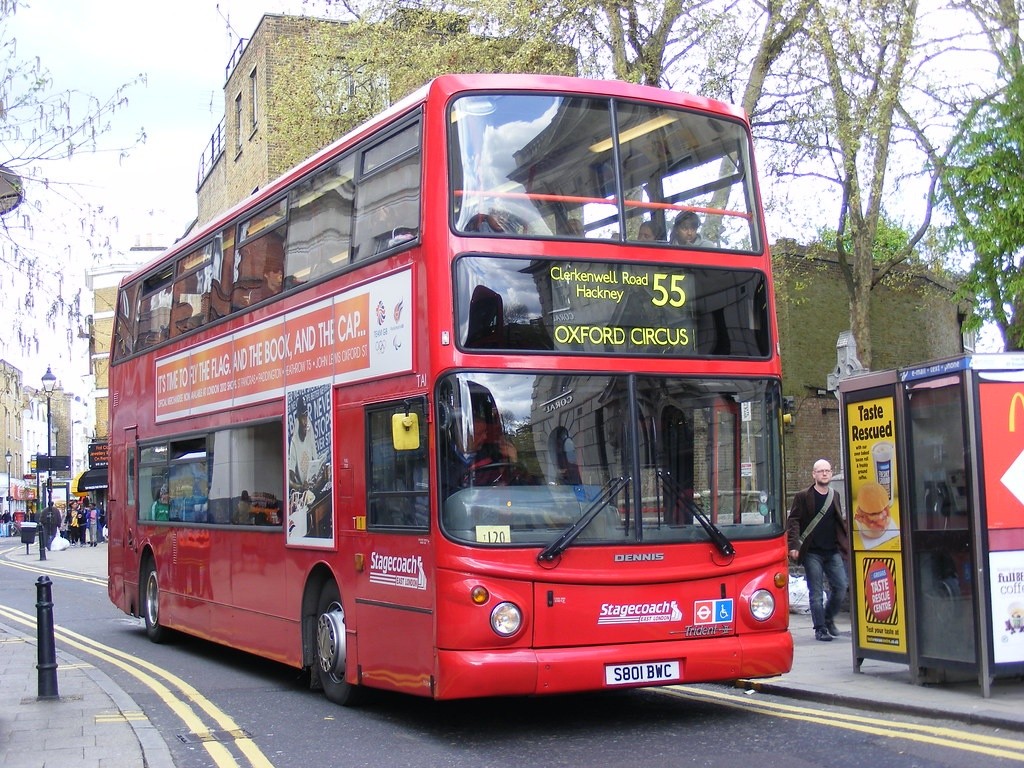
left=94, top=542, right=97, bottom=548
left=89, top=542, right=93, bottom=547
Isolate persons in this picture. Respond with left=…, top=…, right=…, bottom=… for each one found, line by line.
left=81, top=503, right=105, bottom=546
left=289, top=399, right=329, bottom=496
left=467, top=204, right=515, bottom=234
left=248, top=257, right=283, bottom=303
left=669, top=211, right=715, bottom=248
left=59, top=502, right=82, bottom=546
left=638, top=222, right=664, bottom=243
left=562, top=219, right=584, bottom=238
left=40, top=501, right=61, bottom=551
left=787, top=460, right=846, bottom=641
left=445, top=415, right=520, bottom=498
left=148, top=484, right=168, bottom=521
left=2, top=510, right=11, bottom=523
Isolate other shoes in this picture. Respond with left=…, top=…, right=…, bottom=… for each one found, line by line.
left=815, top=629, right=832, bottom=641
left=824, top=622, right=841, bottom=636
left=47, top=544, right=51, bottom=551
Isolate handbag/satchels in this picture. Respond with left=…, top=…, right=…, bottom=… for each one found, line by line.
left=788, top=536, right=811, bottom=567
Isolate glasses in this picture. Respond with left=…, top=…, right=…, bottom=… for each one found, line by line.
left=813, top=470, right=832, bottom=474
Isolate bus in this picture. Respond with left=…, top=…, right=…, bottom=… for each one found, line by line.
left=109, top=70, right=794, bottom=708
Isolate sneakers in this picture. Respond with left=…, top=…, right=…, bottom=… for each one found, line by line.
left=72, top=541, right=78, bottom=546
left=80, top=543, right=86, bottom=547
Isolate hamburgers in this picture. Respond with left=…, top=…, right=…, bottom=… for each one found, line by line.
left=854, top=482, right=890, bottom=539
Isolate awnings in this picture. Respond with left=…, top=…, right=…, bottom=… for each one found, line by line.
left=71, top=471, right=88, bottom=496
left=78, top=469, right=108, bottom=492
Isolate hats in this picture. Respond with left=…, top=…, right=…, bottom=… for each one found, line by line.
left=295, top=395, right=308, bottom=419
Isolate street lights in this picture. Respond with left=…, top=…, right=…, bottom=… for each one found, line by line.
left=5, top=450, right=14, bottom=520
left=41, top=367, right=57, bottom=552
